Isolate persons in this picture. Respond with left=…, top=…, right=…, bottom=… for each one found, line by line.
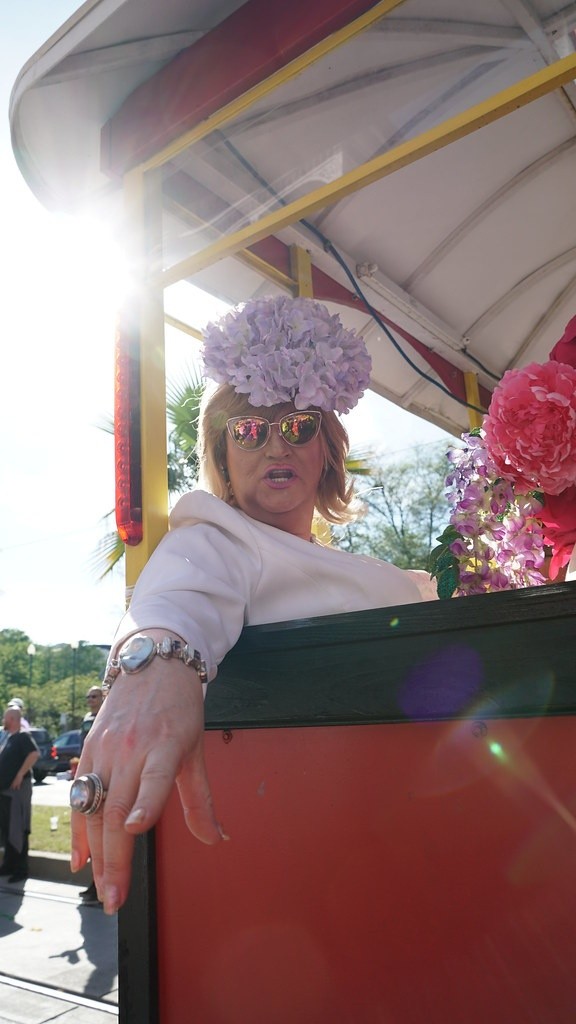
left=59, top=371, right=448, bottom=915
left=1, top=687, right=109, bottom=901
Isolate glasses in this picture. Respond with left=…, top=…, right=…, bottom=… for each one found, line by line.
left=87, top=695, right=97, bottom=699
left=226, top=409, right=321, bottom=452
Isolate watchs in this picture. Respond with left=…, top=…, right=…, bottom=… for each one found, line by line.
left=102, top=633, right=207, bottom=694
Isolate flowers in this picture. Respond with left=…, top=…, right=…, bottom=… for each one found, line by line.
left=203, top=296, right=372, bottom=408
left=436, top=351, right=576, bottom=593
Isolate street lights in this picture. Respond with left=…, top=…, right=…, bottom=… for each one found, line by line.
left=25, top=640, right=35, bottom=727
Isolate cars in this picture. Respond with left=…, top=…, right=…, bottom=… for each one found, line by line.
left=43, top=729, right=86, bottom=773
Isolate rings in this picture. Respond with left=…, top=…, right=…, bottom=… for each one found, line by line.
left=71, top=773, right=109, bottom=817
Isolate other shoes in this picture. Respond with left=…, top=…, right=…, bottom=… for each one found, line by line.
left=80, top=883, right=98, bottom=896
left=83, top=892, right=101, bottom=905
left=9, top=872, right=28, bottom=883
left=0, top=865, right=15, bottom=876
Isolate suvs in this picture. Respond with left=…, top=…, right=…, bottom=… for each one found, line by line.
left=17, top=727, right=58, bottom=784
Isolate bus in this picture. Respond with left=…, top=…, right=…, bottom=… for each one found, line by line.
left=7, top=0, right=576, bottom=1024
